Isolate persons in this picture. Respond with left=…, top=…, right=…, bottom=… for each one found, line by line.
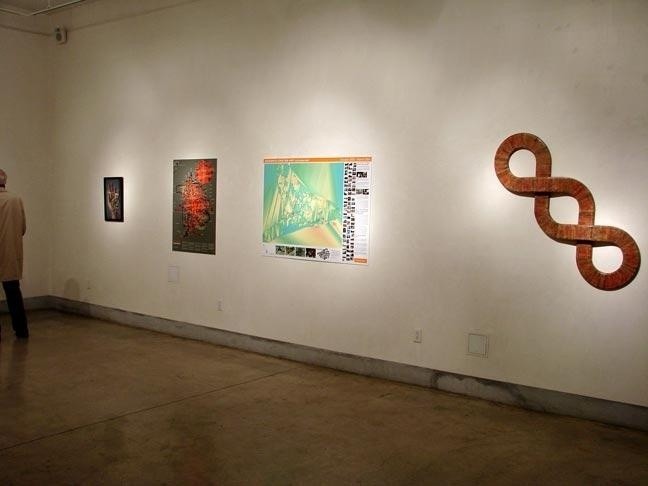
left=0, top=169, right=29, bottom=340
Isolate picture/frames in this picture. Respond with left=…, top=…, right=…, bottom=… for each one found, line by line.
left=102, top=175, right=126, bottom=223
